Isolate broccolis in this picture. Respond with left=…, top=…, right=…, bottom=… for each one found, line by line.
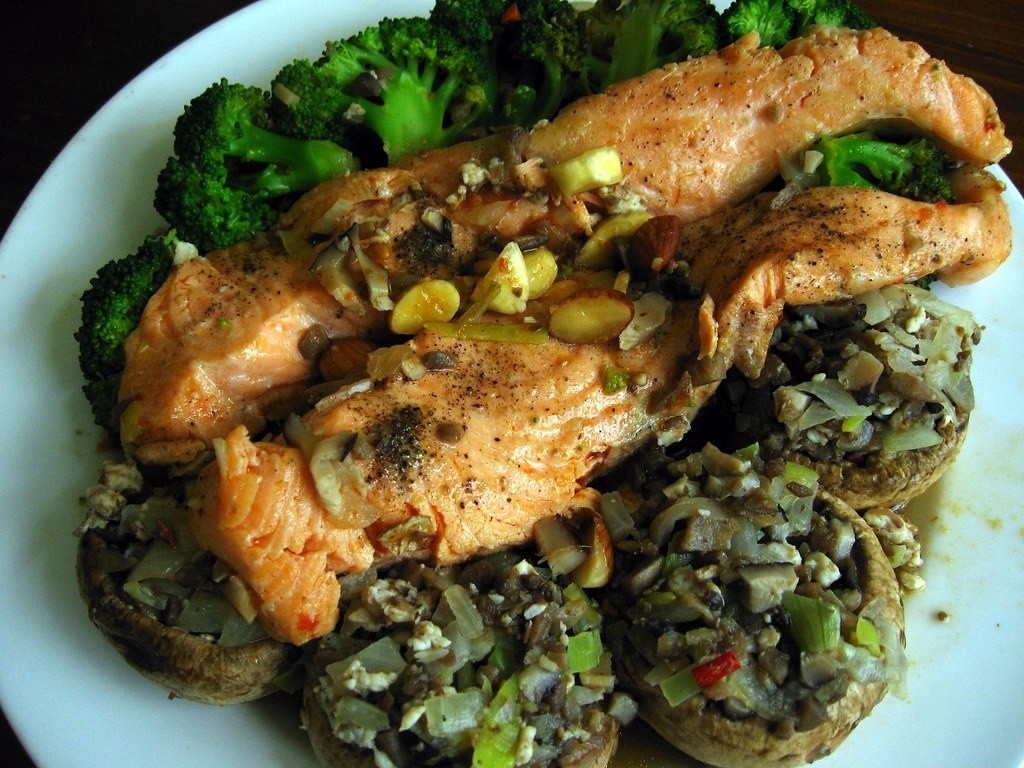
left=72, top=0, right=960, bottom=442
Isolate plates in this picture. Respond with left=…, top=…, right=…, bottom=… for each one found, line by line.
left=0, top=0, right=1024, bottom=768
